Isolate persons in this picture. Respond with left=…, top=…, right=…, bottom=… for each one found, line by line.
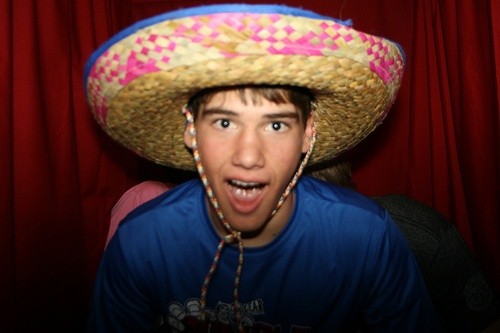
left=88, top=6, right=416, bottom=333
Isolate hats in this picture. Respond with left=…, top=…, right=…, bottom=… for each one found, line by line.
left=83, top=3, right=405, bottom=172
